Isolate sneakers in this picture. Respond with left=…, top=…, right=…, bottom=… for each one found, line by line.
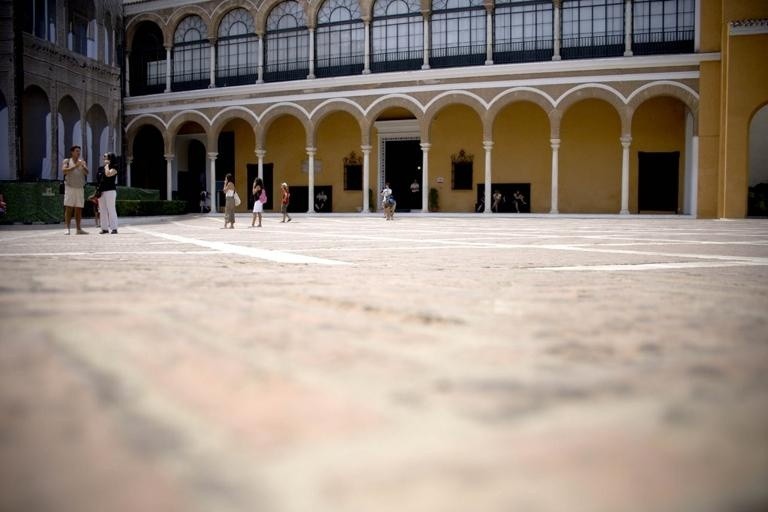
left=76, top=228, right=89, bottom=234
left=99, top=230, right=109, bottom=234
left=63, top=228, right=70, bottom=235
left=111, top=229, right=118, bottom=234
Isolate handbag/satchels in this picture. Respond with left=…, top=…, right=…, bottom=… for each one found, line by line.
left=233, top=190, right=242, bottom=207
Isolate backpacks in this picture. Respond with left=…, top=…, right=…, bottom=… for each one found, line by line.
left=259, top=188, right=268, bottom=204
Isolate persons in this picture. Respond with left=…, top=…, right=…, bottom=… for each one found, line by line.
left=87, top=184, right=101, bottom=228
left=512, top=189, right=527, bottom=213
left=61, top=144, right=89, bottom=235
left=384, top=197, right=397, bottom=221
left=381, top=182, right=393, bottom=217
left=409, top=177, right=420, bottom=208
left=476, top=191, right=485, bottom=212
left=199, top=186, right=209, bottom=213
left=222, top=173, right=235, bottom=229
left=314, top=191, right=328, bottom=212
left=94, top=151, right=119, bottom=234
left=279, top=182, right=291, bottom=223
left=490, top=188, right=502, bottom=212
left=249, top=177, right=263, bottom=227
